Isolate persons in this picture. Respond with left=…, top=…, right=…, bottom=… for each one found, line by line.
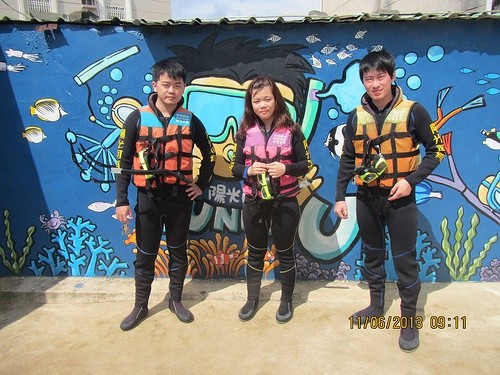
left=229, top=76, right=313, bottom=324
left=334, top=49, right=446, bottom=353
left=115, top=58, right=216, bottom=332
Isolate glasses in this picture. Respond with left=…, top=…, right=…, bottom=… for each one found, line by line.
left=138, top=147, right=156, bottom=180
left=257, top=171, right=275, bottom=200
left=355, top=153, right=388, bottom=184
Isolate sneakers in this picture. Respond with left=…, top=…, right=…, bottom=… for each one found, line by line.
left=168, top=297, right=194, bottom=323
left=238, top=297, right=258, bottom=320
left=399, top=308, right=420, bottom=353
left=120, top=304, right=148, bottom=332
left=276, top=297, right=293, bottom=324
left=351, top=305, right=384, bottom=326
